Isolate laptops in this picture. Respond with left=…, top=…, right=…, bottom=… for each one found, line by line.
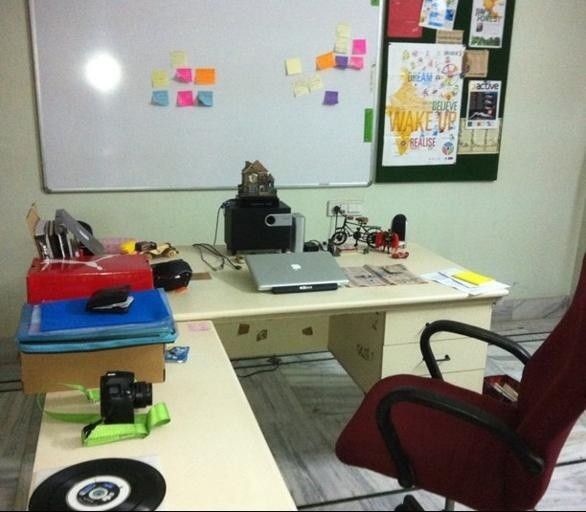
left=244, top=251, right=350, bottom=292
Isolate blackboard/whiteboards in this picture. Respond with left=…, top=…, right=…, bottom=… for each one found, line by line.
left=27, top=0, right=385, bottom=194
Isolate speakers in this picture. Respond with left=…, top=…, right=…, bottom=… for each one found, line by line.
left=391, top=214, right=408, bottom=248
left=150, top=259, right=192, bottom=292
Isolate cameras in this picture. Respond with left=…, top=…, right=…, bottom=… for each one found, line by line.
left=100, top=370, right=152, bottom=424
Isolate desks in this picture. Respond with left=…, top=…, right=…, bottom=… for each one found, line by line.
left=24, top=319, right=296, bottom=511
left=157, top=245, right=513, bottom=397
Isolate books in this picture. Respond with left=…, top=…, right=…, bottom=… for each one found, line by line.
left=453, top=270, right=495, bottom=286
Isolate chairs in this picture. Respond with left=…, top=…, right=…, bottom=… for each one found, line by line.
left=335, top=252, right=586, bottom=512
